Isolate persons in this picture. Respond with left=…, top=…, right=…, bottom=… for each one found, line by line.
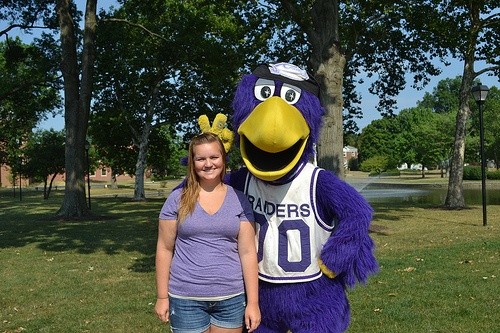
left=155, top=132, right=261, bottom=333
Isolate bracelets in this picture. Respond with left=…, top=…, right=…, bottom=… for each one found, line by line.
left=157, top=297, right=169, bottom=299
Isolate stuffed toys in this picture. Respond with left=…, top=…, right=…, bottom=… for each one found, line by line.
left=172, top=63, right=378, bottom=333
